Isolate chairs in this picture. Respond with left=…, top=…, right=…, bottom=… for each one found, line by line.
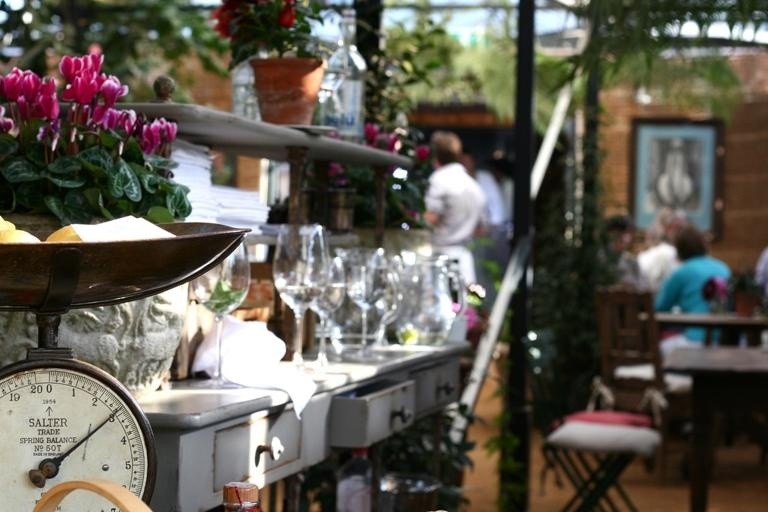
left=516, top=283, right=767, bottom=512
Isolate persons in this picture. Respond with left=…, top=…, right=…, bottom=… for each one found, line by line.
left=647, top=137, right=695, bottom=206
left=635, top=208, right=689, bottom=312
left=606, top=214, right=639, bottom=283
left=420, top=125, right=482, bottom=294
left=486, top=148, right=516, bottom=243
left=750, top=243, right=767, bottom=299
left=652, top=225, right=732, bottom=354
left=460, top=147, right=510, bottom=265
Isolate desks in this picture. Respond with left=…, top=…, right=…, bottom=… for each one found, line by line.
left=139, top=340, right=479, bottom=511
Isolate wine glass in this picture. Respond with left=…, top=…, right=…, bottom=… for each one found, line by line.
left=318, top=66, right=348, bottom=143
left=191, top=241, right=243, bottom=389
left=312, top=249, right=468, bottom=365
left=276, top=224, right=331, bottom=363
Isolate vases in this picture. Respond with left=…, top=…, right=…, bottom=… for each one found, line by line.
left=246, top=52, right=321, bottom=127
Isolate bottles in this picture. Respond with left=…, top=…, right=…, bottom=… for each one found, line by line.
left=230, top=62, right=259, bottom=120
left=222, top=498, right=262, bottom=511
left=337, top=448, right=372, bottom=510
left=328, top=10, right=368, bottom=141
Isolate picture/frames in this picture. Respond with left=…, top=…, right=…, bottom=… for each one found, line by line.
left=623, top=113, right=729, bottom=249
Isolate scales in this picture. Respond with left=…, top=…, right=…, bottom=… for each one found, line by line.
left=0, top=215, right=252, bottom=512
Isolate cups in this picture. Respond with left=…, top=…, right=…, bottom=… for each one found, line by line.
left=382, top=471, right=443, bottom=509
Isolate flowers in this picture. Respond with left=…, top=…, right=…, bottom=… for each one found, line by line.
left=1, top=46, right=191, bottom=229
left=212, top=0, right=347, bottom=73
left=311, top=116, right=436, bottom=236
